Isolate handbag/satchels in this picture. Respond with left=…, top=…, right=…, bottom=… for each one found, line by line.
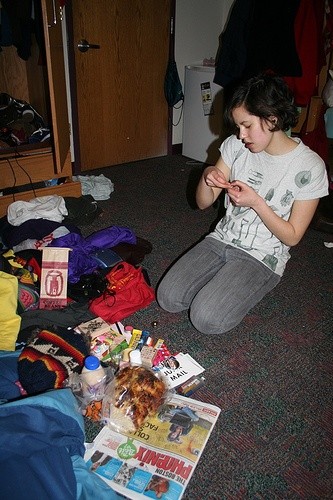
left=87, top=262, right=155, bottom=325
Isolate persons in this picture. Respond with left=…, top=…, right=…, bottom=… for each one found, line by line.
left=85, top=401, right=199, bottom=500
left=154, top=74, right=330, bottom=335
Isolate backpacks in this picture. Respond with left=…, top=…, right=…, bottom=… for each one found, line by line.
left=163, top=56, right=184, bottom=127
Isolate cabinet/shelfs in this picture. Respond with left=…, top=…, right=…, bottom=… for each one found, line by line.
left=1, top=148, right=82, bottom=218
left=0, top=0, right=71, bottom=173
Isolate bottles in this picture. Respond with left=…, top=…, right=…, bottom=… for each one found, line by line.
left=79, top=356, right=105, bottom=398
